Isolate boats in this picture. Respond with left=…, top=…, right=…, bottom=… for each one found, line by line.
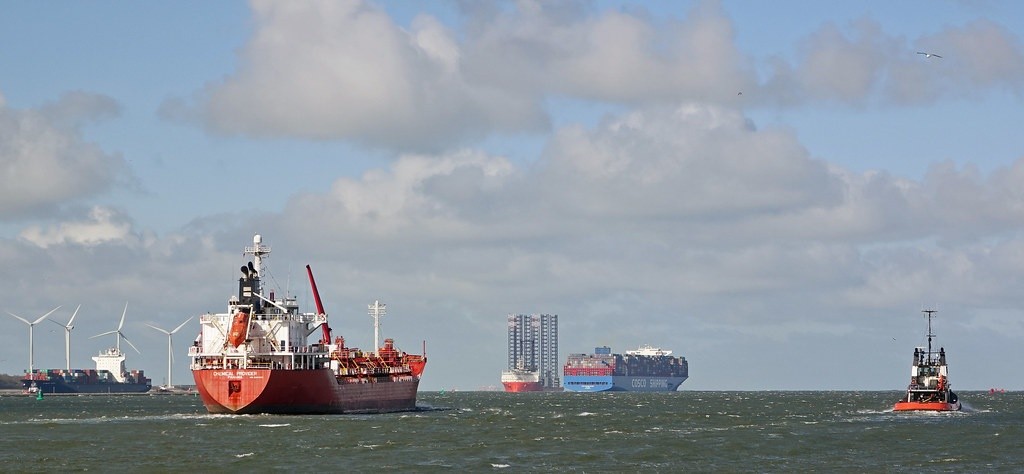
left=501, top=357, right=544, bottom=392
left=562, top=345, right=689, bottom=393
left=188, top=233, right=427, bottom=415
left=229, top=311, right=249, bottom=350
left=894, top=307, right=962, bottom=412
left=19, top=346, right=151, bottom=396
